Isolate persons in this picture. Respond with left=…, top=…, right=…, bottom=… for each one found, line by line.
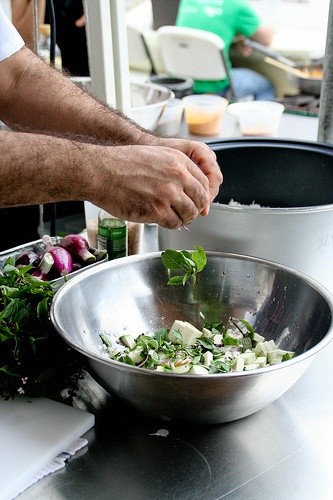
left=0, top=0, right=223, bottom=229
left=174, top=0, right=275, bottom=102
left=0, top=0, right=91, bottom=77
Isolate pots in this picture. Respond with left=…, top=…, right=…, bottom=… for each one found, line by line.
left=158, top=139, right=333, bottom=294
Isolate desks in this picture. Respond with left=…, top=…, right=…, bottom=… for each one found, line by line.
left=244, top=40, right=326, bottom=100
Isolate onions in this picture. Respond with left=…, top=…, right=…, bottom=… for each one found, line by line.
left=14, top=234, right=97, bottom=282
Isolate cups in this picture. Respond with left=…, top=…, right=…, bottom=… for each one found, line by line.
left=232, top=102, right=284, bottom=136
left=183, top=96, right=225, bottom=136
left=148, top=76, right=192, bottom=96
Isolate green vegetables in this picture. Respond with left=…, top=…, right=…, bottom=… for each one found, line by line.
left=96, top=243, right=255, bottom=375
left=0, top=257, right=75, bottom=389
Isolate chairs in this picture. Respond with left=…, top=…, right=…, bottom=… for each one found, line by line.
left=126, top=22, right=159, bottom=77
left=153, top=25, right=255, bottom=103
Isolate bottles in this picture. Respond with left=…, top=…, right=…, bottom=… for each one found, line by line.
left=97, top=208, right=127, bottom=255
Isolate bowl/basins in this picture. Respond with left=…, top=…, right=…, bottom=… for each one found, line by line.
left=68, top=79, right=173, bottom=133
left=52, top=250, right=333, bottom=423
left=291, top=64, right=322, bottom=96
